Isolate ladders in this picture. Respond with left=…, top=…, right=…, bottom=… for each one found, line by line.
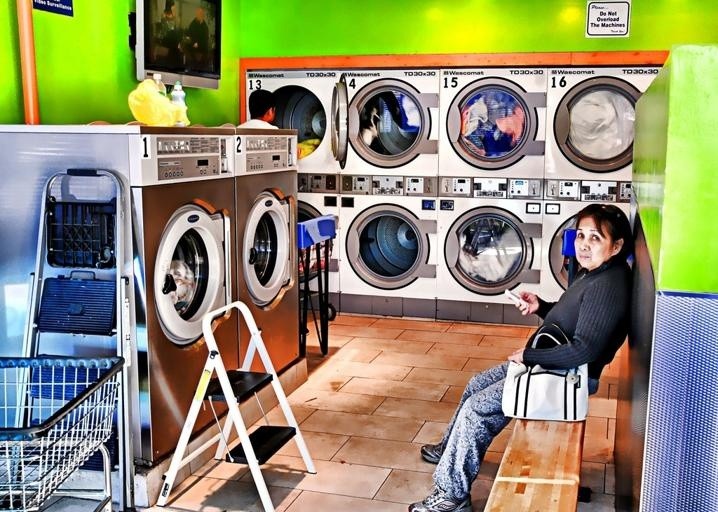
left=155, top=301, right=318, bottom=512
left=9, top=168, right=143, bottom=512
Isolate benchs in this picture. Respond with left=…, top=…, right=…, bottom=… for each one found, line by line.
left=483, top=418, right=586, bottom=512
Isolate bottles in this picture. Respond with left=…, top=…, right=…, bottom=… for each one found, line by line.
left=152, top=72, right=166, bottom=96
left=168, top=79, right=187, bottom=128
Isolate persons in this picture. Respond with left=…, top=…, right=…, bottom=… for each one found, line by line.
left=158, top=0, right=210, bottom=68
left=236, top=91, right=278, bottom=130
left=406, top=202, right=633, bottom=512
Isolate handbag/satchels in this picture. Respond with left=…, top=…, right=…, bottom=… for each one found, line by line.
left=502, top=322, right=589, bottom=422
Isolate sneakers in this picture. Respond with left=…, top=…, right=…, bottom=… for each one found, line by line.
left=408, top=489, right=472, bottom=512
left=421, top=443, right=446, bottom=463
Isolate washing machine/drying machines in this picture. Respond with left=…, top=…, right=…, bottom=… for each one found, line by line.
left=1, top=125, right=239, bottom=475
left=438, top=67, right=665, bottom=304
left=234, top=124, right=302, bottom=415
left=244, top=67, right=440, bottom=303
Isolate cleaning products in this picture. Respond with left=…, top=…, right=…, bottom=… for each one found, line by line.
left=150, top=74, right=190, bottom=126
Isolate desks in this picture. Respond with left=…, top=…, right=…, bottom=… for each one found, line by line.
left=296, top=213, right=338, bottom=358
